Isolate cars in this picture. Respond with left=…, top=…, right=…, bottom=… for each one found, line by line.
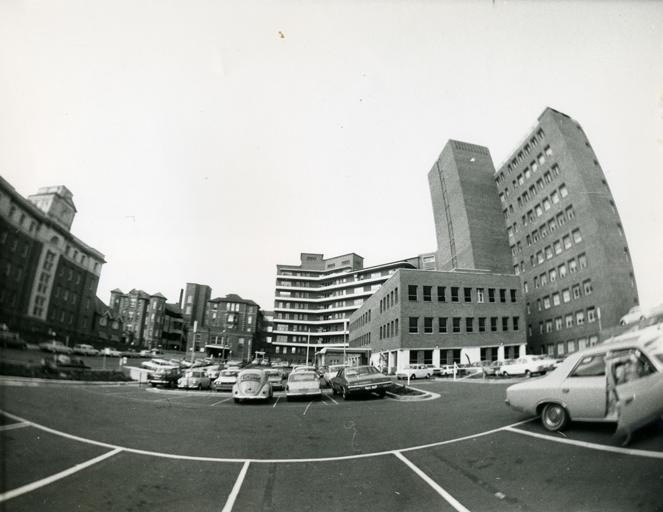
left=283, top=370, right=323, bottom=402
left=231, top=368, right=275, bottom=404
left=504, top=330, right=663, bottom=441
left=329, top=364, right=393, bottom=399
left=455, top=355, right=562, bottom=377
left=620, top=306, right=662, bottom=326
left=395, top=361, right=462, bottom=379
